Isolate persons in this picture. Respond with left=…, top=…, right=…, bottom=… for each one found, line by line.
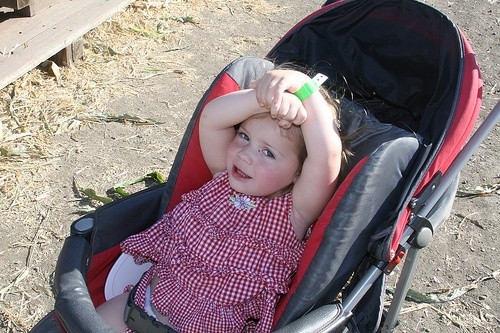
left=96, top=66, right=343, bottom=329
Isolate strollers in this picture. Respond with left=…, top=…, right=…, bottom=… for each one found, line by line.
left=31, top=0, right=500, bottom=332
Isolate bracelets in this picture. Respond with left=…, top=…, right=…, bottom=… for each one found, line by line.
left=291, top=70, right=329, bottom=98
